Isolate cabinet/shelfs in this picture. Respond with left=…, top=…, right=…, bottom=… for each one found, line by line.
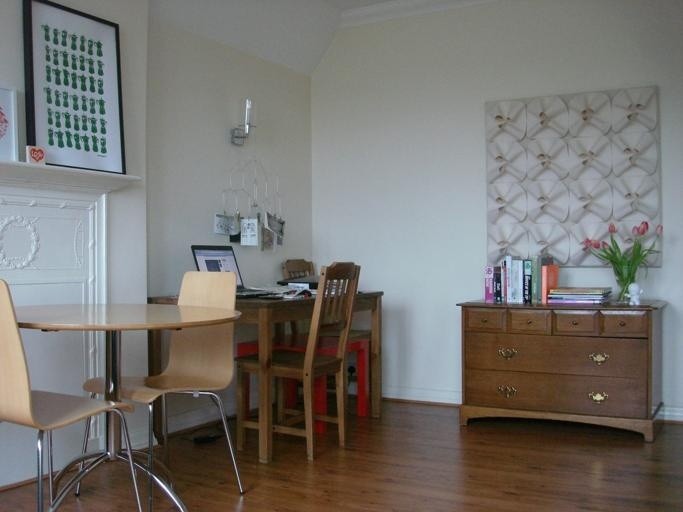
left=457, top=299, right=668, bottom=443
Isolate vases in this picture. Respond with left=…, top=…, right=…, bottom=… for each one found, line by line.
left=612, top=265, right=639, bottom=306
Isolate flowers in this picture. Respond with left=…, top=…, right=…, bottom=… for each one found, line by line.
left=579, top=221, right=663, bottom=301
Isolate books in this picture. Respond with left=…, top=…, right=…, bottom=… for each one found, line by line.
left=214, top=211, right=287, bottom=253
left=276, top=275, right=341, bottom=295
left=484, top=254, right=613, bottom=305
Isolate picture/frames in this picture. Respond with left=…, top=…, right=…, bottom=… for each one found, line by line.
left=23, top=0, right=126, bottom=175
left=0, top=86, right=19, bottom=161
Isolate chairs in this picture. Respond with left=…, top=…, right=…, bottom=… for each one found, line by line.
left=282, top=258, right=371, bottom=383
left=0, top=279, right=143, bottom=512
left=75, top=271, right=244, bottom=497
left=235, top=262, right=360, bottom=462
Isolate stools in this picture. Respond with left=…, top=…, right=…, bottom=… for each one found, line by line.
left=237, top=330, right=367, bottom=439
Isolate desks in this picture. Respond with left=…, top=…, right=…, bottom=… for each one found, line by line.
left=148, top=292, right=384, bottom=465
left=14, top=304, right=243, bottom=512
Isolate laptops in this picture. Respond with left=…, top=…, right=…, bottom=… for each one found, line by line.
left=192, top=245, right=279, bottom=297
